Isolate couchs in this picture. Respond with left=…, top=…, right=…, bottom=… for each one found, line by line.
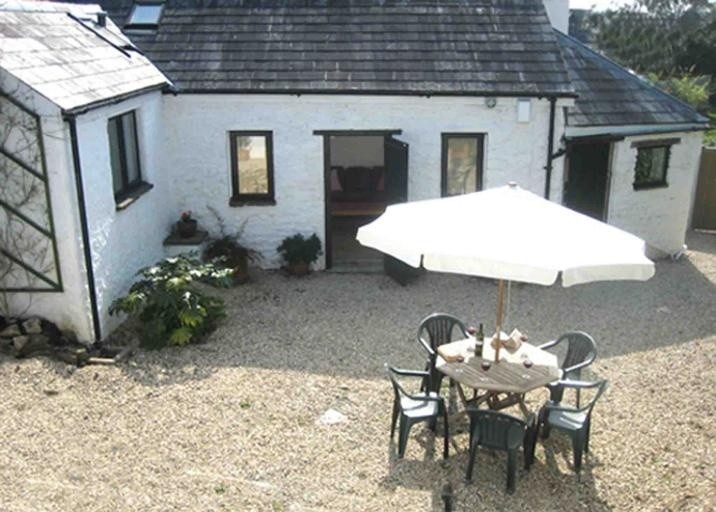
left=330, top=164, right=387, bottom=217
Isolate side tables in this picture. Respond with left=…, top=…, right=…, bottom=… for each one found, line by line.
left=434, top=335, right=560, bottom=427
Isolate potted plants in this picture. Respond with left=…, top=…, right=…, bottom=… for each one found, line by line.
left=276, top=231, right=324, bottom=275
left=203, top=202, right=266, bottom=287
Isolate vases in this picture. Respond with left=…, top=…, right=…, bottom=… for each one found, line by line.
left=176, top=220, right=198, bottom=238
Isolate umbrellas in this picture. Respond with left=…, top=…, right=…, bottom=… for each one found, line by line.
left=355, top=181, right=658, bottom=365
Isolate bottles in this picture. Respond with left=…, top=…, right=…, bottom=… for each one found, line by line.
left=475, top=323, right=485, bottom=356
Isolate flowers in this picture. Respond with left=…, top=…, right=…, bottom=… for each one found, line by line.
left=179, top=208, right=193, bottom=222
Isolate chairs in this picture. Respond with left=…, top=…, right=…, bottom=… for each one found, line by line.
left=536, top=330, right=599, bottom=407
left=386, top=365, right=450, bottom=460
left=463, top=409, right=533, bottom=492
left=416, top=312, right=473, bottom=392
left=534, top=380, right=609, bottom=473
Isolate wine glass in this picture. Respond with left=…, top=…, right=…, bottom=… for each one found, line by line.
left=455, top=353, right=464, bottom=373
left=464, top=319, right=479, bottom=352
left=480, top=362, right=491, bottom=382
left=522, top=360, right=533, bottom=380
